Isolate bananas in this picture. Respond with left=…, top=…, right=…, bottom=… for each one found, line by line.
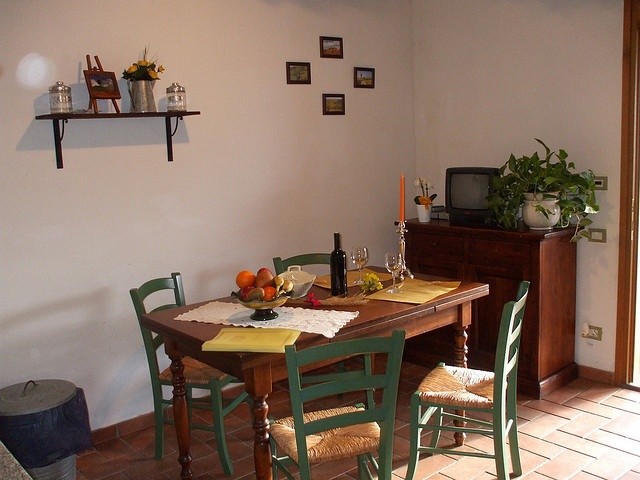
left=273, top=275, right=292, bottom=298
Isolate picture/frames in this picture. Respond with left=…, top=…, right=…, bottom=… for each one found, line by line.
left=320, top=36, right=343, bottom=59
left=322, top=93, right=346, bottom=115
left=83, top=70, right=121, bottom=99
left=353, top=67, right=375, bottom=89
left=285, top=62, right=311, bottom=84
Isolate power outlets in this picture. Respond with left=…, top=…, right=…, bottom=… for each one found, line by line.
left=582, top=325, right=602, bottom=341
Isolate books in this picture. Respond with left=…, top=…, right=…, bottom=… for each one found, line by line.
left=202, top=327, right=300, bottom=353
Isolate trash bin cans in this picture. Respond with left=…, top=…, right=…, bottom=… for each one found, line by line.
left=0, top=379, right=78, bottom=479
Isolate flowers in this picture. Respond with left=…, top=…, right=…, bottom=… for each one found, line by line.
left=122, top=46, right=164, bottom=79
left=412, top=176, right=438, bottom=210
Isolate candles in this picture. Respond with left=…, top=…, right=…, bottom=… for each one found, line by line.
left=398, top=172, right=406, bottom=221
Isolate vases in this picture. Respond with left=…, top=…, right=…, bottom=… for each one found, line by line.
left=416, top=205, right=432, bottom=223
left=126, top=79, right=157, bottom=112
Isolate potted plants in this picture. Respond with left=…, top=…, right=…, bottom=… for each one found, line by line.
left=483, top=138, right=601, bottom=243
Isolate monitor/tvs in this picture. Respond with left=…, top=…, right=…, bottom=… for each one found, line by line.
left=445, top=167, right=502, bottom=227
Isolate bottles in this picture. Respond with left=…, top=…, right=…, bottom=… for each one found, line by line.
left=330, top=232, right=348, bottom=298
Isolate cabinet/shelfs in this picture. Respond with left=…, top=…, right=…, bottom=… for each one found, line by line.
left=394, top=217, right=586, bottom=400
left=35, top=111, right=201, bottom=169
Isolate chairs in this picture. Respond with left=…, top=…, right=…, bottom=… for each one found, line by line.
left=273, top=252, right=376, bottom=411
left=404, top=281, right=531, bottom=480
left=268, top=328, right=407, bottom=480
left=128, top=272, right=253, bottom=476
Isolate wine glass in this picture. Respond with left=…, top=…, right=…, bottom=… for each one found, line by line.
left=385, top=253, right=402, bottom=294
left=351, top=247, right=369, bottom=284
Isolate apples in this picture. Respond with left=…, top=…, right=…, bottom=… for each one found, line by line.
left=254, top=267, right=273, bottom=286
left=246, top=288, right=265, bottom=303
left=238, top=284, right=256, bottom=304
